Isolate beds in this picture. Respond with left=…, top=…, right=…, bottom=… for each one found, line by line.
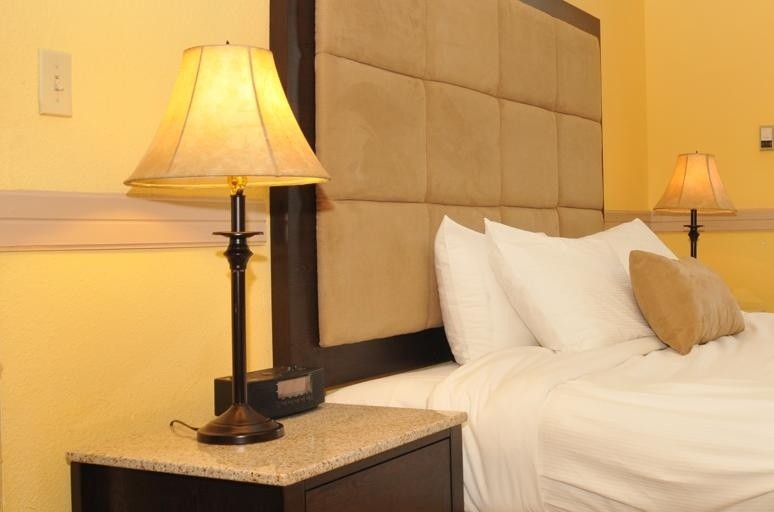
left=320, top=313, right=774, bottom=512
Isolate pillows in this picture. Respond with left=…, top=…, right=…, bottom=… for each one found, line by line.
left=482, top=215, right=679, bottom=355
left=628, top=250, right=745, bottom=355
left=435, top=213, right=546, bottom=366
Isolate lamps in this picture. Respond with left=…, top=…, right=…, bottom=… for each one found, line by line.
left=122, top=43, right=331, bottom=444
left=657, top=153, right=728, bottom=258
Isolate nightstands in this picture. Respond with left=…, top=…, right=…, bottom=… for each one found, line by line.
left=63, top=402, right=466, bottom=512
